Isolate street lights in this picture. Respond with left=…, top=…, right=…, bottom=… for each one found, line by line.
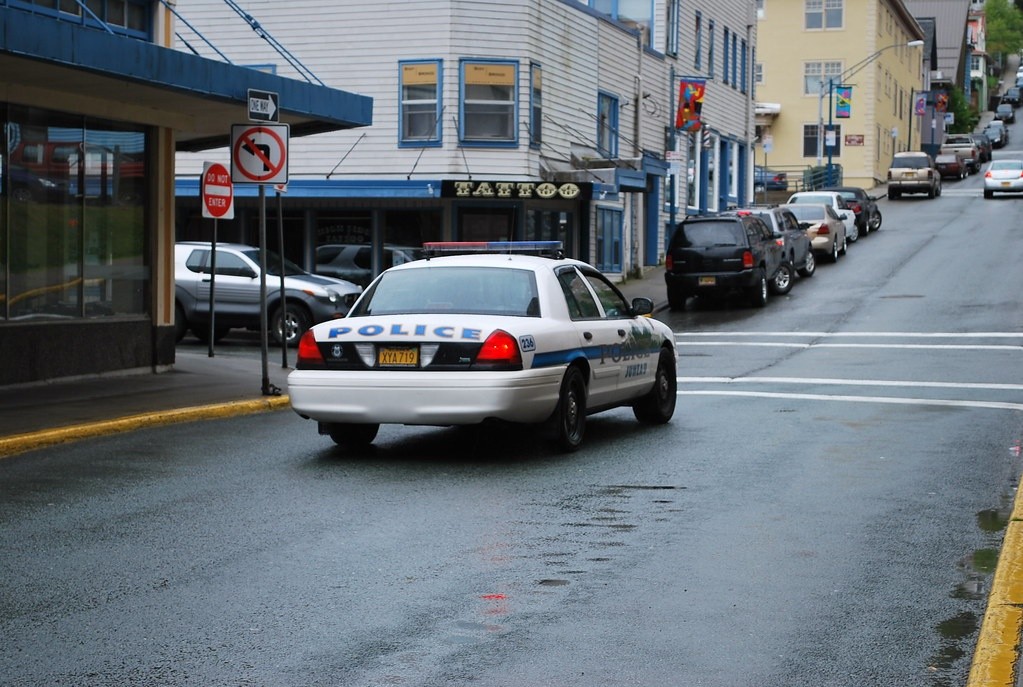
left=814, top=39, right=926, bottom=186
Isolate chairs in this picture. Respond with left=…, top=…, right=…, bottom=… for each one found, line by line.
left=458, top=281, right=495, bottom=310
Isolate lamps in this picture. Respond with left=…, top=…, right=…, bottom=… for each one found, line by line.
left=428, top=183, right=435, bottom=194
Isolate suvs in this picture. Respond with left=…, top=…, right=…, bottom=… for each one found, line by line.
left=174, top=240, right=365, bottom=349
left=721, top=202, right=817, bottom=280
left=311, top=241, right=424, bottom=295
left=664, top=211, right=796, bottom=312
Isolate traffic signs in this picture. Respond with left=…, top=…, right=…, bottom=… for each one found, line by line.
left=247, top=88, right=280, bottom=123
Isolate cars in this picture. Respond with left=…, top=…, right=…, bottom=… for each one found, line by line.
left=786, top=190, right=861, bottom=245
left=822, top=186, right=883, bottom=236
left=286, top=238, right=678, bottom=455
left=983, top=158, right=1023, bottom=199
left=775, top=201, right=848, bottom=262
left=1, top=140, right=145, bottom=207
left=754, top=164, right=789, bottom=195
left=936, top=65, right=1023, bottom=181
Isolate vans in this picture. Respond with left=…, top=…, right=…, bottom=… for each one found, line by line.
left=887, top=151, right=942, bottom=200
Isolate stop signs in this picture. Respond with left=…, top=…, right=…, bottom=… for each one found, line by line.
left=201, top=161, right=235, bottom=219
